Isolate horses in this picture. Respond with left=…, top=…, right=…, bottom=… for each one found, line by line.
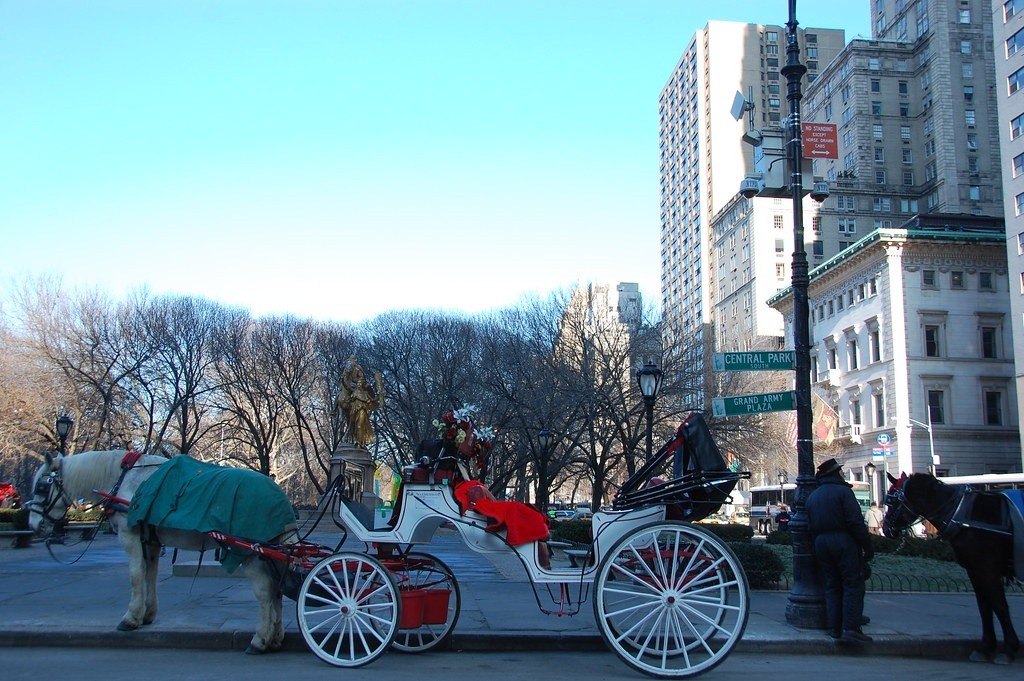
left=881, top=468, right=1024, bottom=665
left=26, top=451, right=298, bottom=655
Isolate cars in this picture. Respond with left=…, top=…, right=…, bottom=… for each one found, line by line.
left=691, top=513, right=725, bottom=524
left=576, top=508, right=594, bottom=518
left=727, top=507, right=750, bottom=524
left=555, top=511, right=575, bottom=518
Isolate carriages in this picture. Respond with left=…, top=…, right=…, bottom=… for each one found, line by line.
left=27, top=411, right=760, bottom=681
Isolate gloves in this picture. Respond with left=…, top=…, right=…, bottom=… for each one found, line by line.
left=864, top=544, right=874, bottom=561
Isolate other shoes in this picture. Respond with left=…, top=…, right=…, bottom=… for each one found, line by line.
left=830, top=627, right=838, bottom=637
left=842, top=629, right=873, bottom=642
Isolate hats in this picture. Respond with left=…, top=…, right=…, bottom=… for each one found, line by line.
left=817, top=458, right=843, bottom=479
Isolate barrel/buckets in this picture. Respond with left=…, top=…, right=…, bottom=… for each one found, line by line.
left=423, top=588, right=451, bottom=624
left=387, top=590, right=427, bottom=629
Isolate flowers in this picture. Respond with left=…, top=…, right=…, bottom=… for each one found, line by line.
left=432, top=403, right=495, bottom=450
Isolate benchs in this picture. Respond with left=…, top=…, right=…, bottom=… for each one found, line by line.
left=467, top=485, right=550, bottom=541
left=607, top=557, right=642, bottom=581
left=0, top=530, right=34, bottom=549
left=546, top=541, right=572, bottom=558
left=637, top=476, right=665, bottom=509
left=403, top=412, right=480, bottom=485
left=563, top=550, right=592, bottom=568
left=62, top=525, right=99, bottom=541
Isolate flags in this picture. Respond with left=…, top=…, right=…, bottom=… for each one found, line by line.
left=811, top=394, right=837, bottom=447
left=786, top=412, right=797, bottom=450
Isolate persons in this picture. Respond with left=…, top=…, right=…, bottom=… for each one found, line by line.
left=860, top=563, right=870, bottom=626
left=352, top=380, right=375, bottom=447
left=865, top=502, right=883, bottom=535
left=806, top=459, right=873, bottom=644
left=775, top=506, right=791, bottom=530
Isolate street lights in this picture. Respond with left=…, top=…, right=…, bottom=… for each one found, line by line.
left=778, top=470, right=785, bottom=504
left=637, top=359, right=665, bottom=461
left=889, top=415, right=937, bottom=477
left=55, top=413, right=74, bottom=457
left=865, top=462, right=876, bottom=507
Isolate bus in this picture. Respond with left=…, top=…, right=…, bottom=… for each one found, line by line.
left=749, top=481, right=871, bottom=536
left=924, top=473, right=1024, bottom=540
